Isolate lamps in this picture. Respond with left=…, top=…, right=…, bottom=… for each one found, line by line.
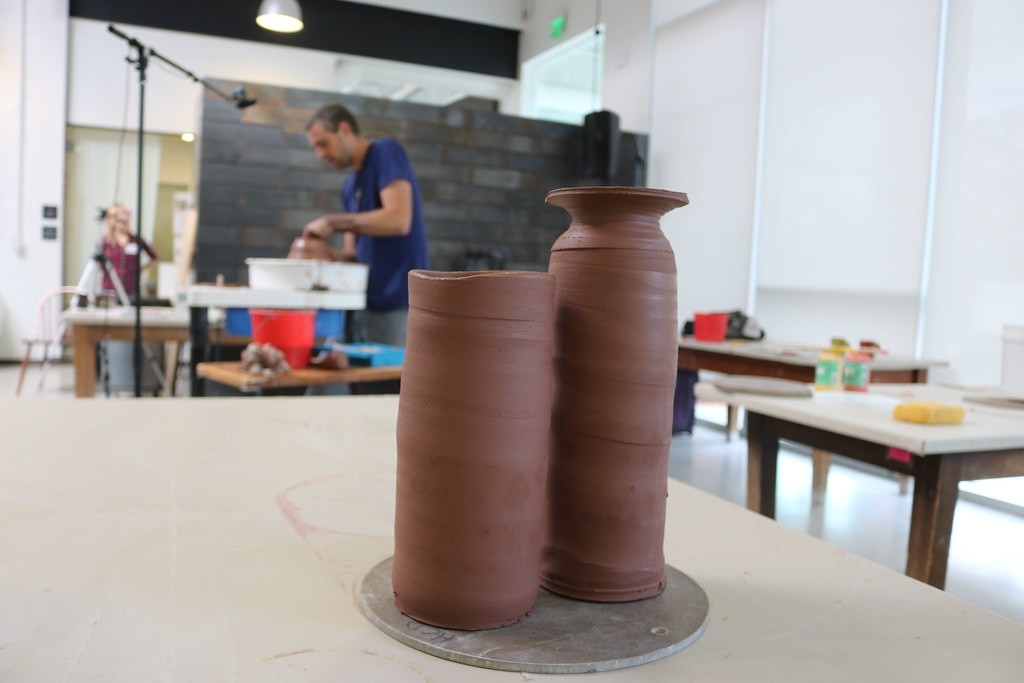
left=255, top=0, right=304, bottom=33
left=106, top=24, right=258, bottom=401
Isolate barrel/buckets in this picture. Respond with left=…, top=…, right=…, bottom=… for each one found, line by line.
left=246, top=308, right=317, bottom=369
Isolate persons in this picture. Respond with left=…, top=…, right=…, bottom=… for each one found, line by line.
left=303, top=103, right=429, bottom=348
left=98, top=205, right=155, bottom=308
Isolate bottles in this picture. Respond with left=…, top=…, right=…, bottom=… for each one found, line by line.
left=842, top=348, right=874, bottom=392
left=544, top=186, right=689, bottom=602
left=814, top=344, right=848, bottom=393
left=831, top=336, right=876, bottom=359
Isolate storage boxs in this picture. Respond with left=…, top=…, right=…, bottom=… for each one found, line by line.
left=224, top=306, right=345, bottom=340
left=244, top=257, right=319, bottom=291
left=315, top=262, right=369, bottom=293
left=692, top=312, right=730, bottom=343
left=312, top=341, right=405, bottom=366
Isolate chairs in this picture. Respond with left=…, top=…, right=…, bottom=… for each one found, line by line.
left=13, top=285, right=97, bottom=396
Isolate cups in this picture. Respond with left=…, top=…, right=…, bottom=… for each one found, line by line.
left=393, top=270, right=557, bottom=631
left=1000, top=320, right=1024, bottom=402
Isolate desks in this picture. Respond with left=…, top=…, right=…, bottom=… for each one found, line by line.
left=677, top=334, right=949, bottom=510
left=192, top=359, right=403, bottom=396
left=0, top=393, right=1024, bottom=683
left=62, top=305, right=254, bottom=399
left=693, top=382, right=1024, bottom=593
